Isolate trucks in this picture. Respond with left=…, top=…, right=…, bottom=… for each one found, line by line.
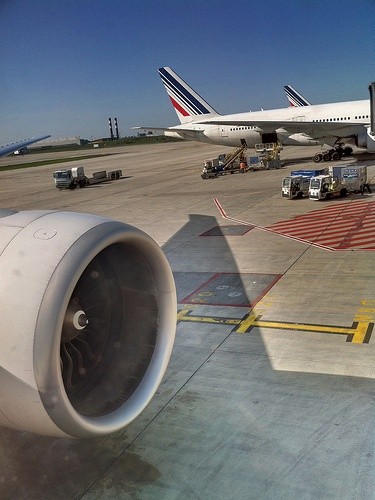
left=52, top=166, right=85, bottom=191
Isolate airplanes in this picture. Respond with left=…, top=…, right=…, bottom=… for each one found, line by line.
left=1, top=135, right=178, bottom=443
left=129, top=66, right=375, bottom=180
